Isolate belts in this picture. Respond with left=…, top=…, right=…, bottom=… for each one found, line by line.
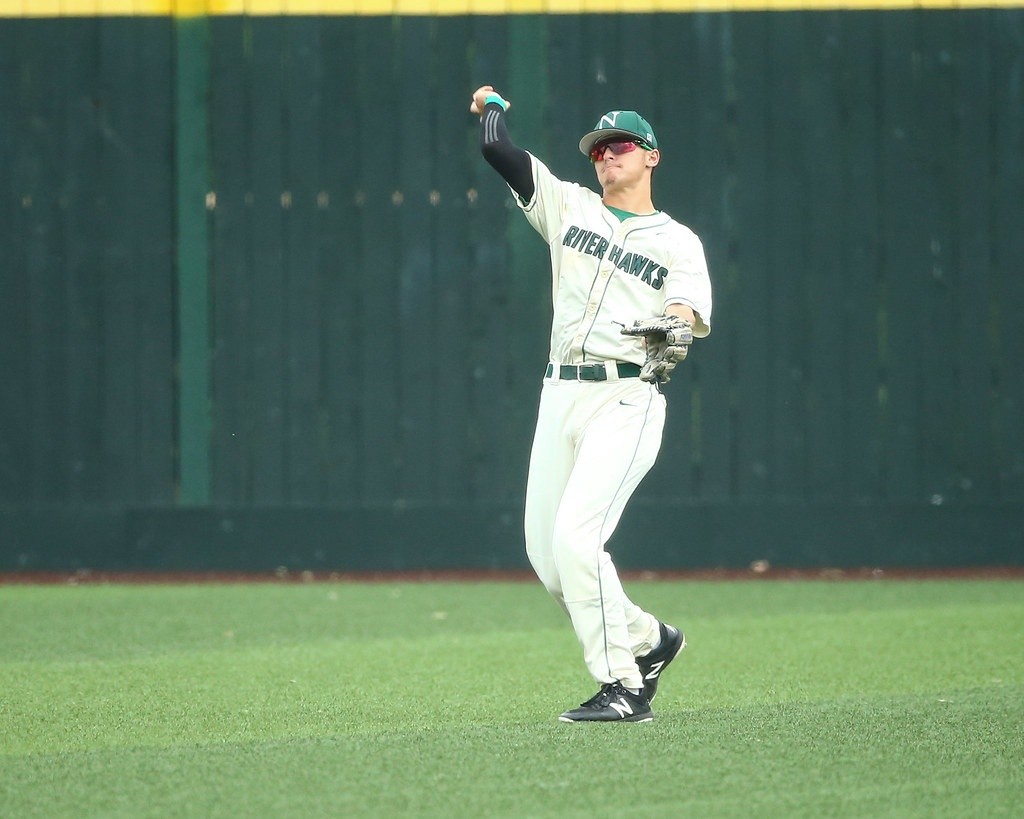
left=545, top=362, right=641, bottom=381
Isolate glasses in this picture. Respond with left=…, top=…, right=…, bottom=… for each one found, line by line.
left=586, top=138, right=639, bottom=163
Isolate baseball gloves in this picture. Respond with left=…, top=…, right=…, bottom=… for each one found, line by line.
left=620, top=316, right=694, bottom=384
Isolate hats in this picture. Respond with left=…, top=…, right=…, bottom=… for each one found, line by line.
left=578, top=110, right=660, bottom=150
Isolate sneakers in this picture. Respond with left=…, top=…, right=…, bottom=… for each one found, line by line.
left=632, top=622, right=687, bottom=705
left=559, top=682, right=656, bottom=723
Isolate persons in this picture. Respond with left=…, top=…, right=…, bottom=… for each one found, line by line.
left=469, top=85, right=713, bottom=722
